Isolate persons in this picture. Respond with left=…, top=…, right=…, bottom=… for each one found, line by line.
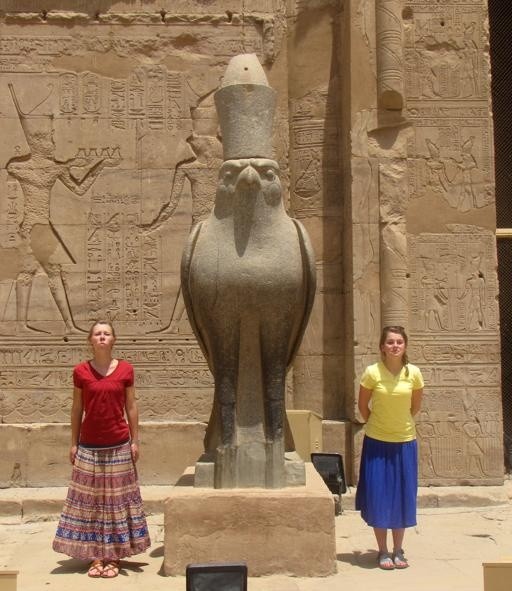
left=52, top=320, right=150, bottom=577
left=354, top=324, right=425, bottom=571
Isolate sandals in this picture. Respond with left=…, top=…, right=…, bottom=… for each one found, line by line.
left=101, top=559, right=121, bottom=578
left=393, top=552, right=408, bottom=568
left=88, top=560, right=104, bottom=577
left=378, top=551, right=396, bottom=569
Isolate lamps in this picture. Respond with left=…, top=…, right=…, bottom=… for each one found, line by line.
left=186, top=563, right=247, bottom=591
left=311, top=453, right=349, bottom=496
